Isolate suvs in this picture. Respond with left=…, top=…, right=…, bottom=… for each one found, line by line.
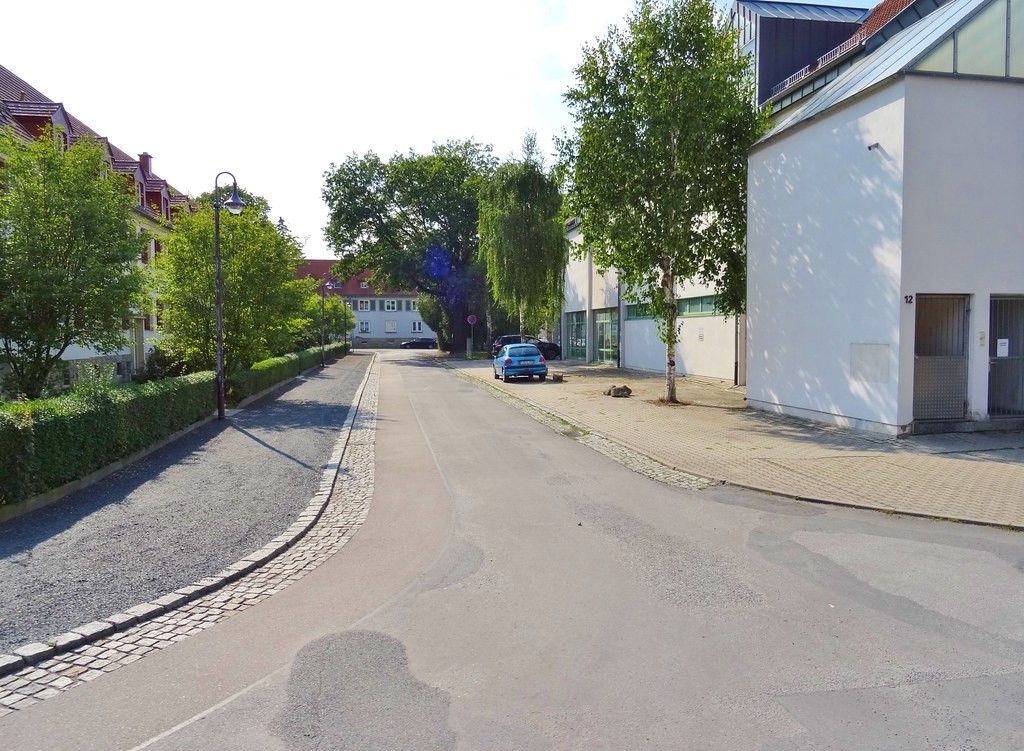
left=400, top=337, right=436, bottom=349
left=491, top=334, right=560, bottom=361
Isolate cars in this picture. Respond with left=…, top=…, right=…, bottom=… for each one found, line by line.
left=492, top=343, right=547, bottom=382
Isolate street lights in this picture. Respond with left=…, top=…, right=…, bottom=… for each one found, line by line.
left=344, top=294, right=353, bottom=357
left=213, top=171, right=247, bottom=421
left=321, top=277, right=333, bottom=369
